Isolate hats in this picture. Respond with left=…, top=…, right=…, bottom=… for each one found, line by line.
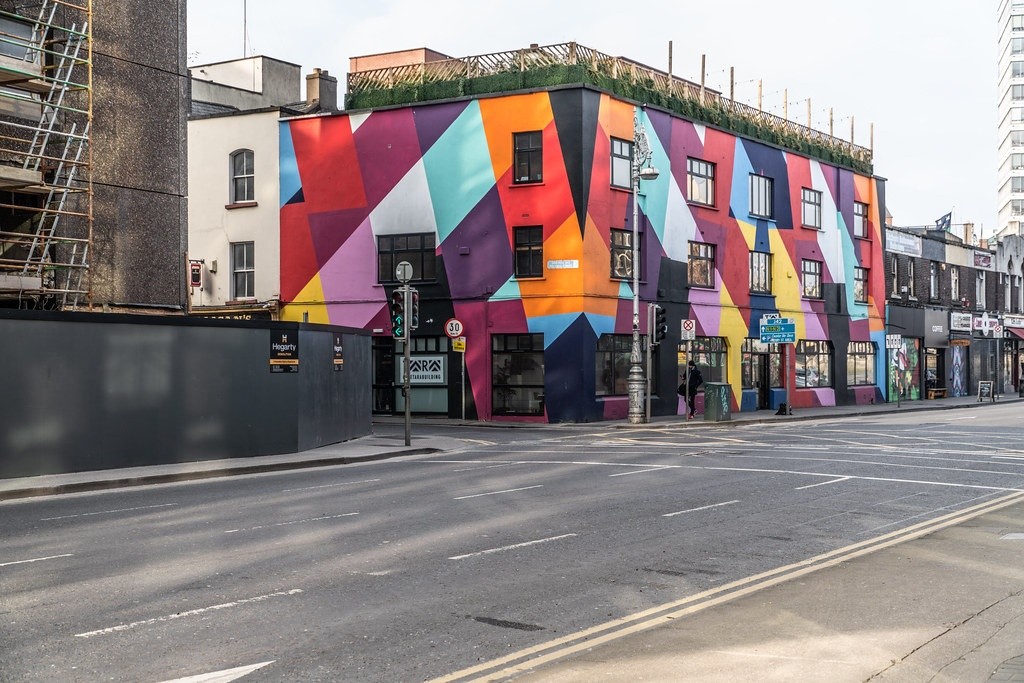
left=689, top=360, right=696, bottom=366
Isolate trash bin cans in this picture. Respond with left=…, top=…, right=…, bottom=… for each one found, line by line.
left=703, top=381, right=733, bottom=421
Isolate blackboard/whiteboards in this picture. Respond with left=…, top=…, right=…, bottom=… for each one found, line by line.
left=978, top=381, right=993, bottom=397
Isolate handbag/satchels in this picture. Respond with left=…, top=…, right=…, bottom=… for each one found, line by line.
left=677, top=382, right=686, bottom=396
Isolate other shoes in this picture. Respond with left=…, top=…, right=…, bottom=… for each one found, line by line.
left=688, top=415, right=694, bottom=421
left=694, top=409, right=698, bottom=415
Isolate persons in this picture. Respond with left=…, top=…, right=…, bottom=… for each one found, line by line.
left=679, top=358, right=703, bottom=419
left=1018, top=348, right=1024, bottom=398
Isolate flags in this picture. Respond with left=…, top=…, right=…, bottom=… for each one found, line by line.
left=936, top=213, right=952, bottom=231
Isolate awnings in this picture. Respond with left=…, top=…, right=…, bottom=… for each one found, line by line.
left=1007, top=327, right=1024, bottom=339
left=190, top=302, right=279, bottom=315
